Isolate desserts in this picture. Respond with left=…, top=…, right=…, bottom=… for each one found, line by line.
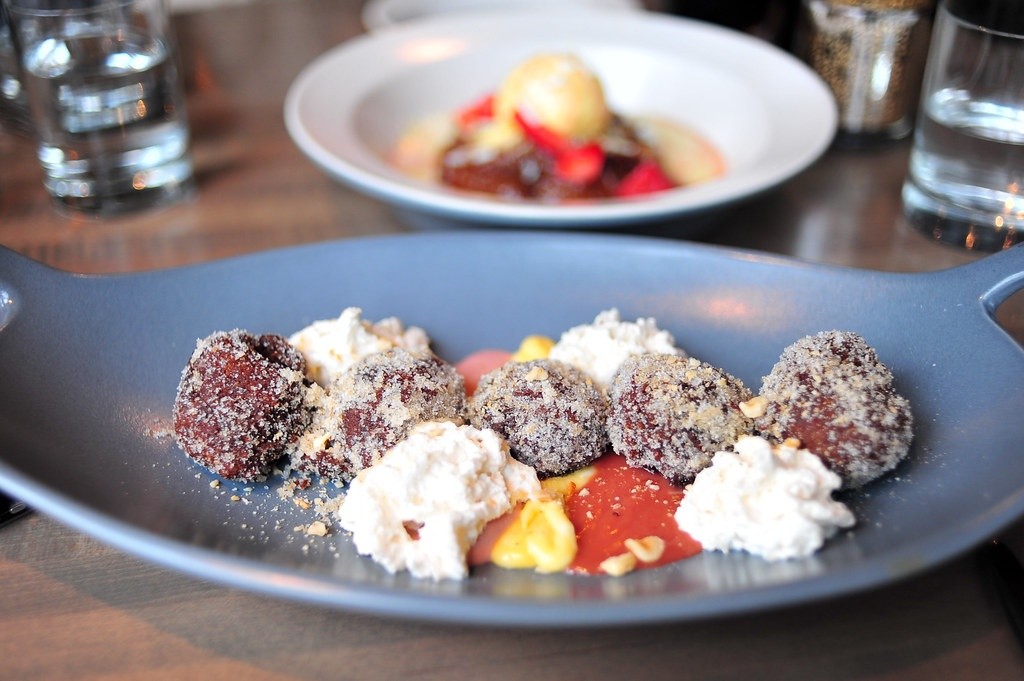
left=168, top=302, right=917, bottom=581
left=389, top=49, right=730, bottom=208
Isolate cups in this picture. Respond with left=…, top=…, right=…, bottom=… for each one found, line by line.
left=802, top=0, right=933, bottom=145
left=13, top=1, right=207, bottom=219
left=901, top=11, right=1024, bottom=247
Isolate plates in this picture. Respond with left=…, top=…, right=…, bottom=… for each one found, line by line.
left=5, top=235, right=1023, bottom=632
left=285, top=8, right=839, bottom=239
